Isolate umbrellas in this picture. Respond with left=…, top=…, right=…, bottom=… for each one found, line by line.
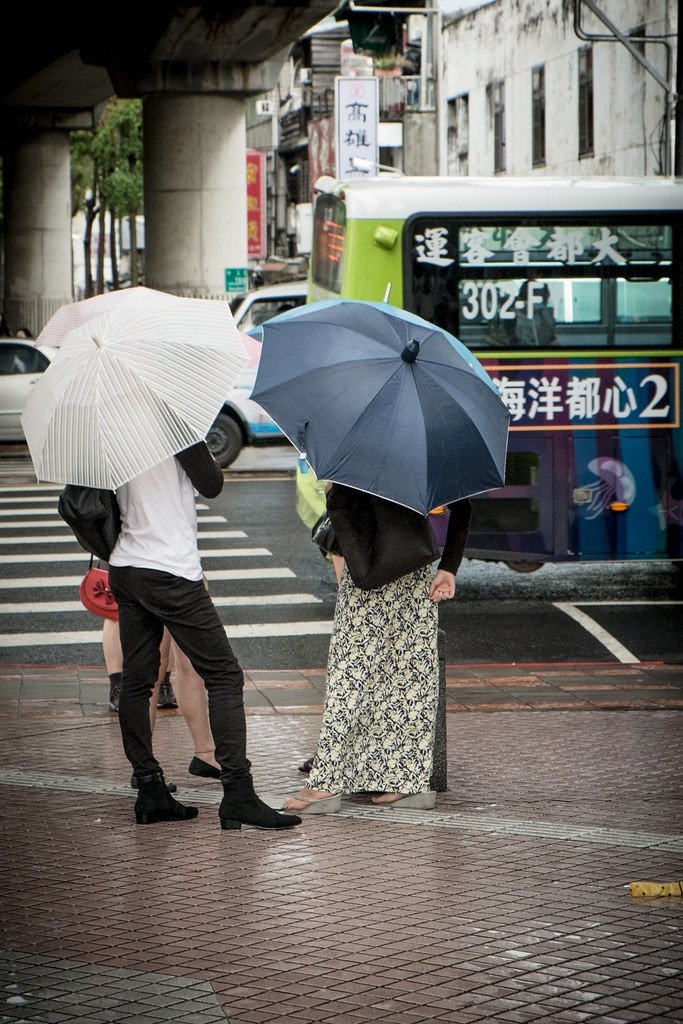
left=251, top=282, right=511, bottom=515
left=18, top=286, right=249, bottom=491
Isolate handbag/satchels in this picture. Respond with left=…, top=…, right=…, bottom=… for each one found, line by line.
left=57, top=483, right=122, bottom=562
left=80, top=566, right=120, bottom=621
left=325, top=483, right=442, bottom=592
left=312, top=515, right=344, bottom=560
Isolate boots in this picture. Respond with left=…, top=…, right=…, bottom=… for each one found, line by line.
left=133, top=766, right=198, bottom=824
left=218, top=774, right=303, bottom=830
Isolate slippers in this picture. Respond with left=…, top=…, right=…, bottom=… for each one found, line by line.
left=381, top=789, right=437, bottom=810
left=284, top=792, right=344, bottom=815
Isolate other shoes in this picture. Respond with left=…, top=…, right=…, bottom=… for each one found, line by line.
left=187, top=755, right=222, bottom=784
left=167, top=782, right=178, bottom=794
left=298, top=758, right=315, bottom=775
left=157, top=680, right=179, bottom=708
left=109, top=683, right=122, bottom=712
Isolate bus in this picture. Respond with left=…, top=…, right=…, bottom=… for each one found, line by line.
left=290, top=165, right=683, bottom=580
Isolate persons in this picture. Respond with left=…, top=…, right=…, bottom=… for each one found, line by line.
left=102, top=435, right=303, bottom=830
left=482, top=281, right=559, bottom=345
left=283, top=481, right=472, bottom=813
left=15, top=328, right=33, bottom=339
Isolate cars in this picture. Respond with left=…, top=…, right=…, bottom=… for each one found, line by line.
left=0, top=338, right=54, bottom=440
left=197, top=283, right=309, bottom=466
